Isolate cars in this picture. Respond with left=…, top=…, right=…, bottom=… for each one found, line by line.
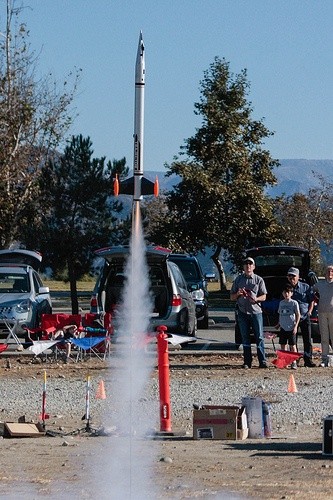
left=0, top=250, right=53, bottom=343
left=92, top=247, right=197, bottom=337
left=234, top=245, right=320, bottom=344
left=308, top=274, right=333, bottom=342
left=166, top=254, right=209, bottom=328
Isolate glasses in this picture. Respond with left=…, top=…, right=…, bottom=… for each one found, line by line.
left=245, top=263, right=253, bottom=265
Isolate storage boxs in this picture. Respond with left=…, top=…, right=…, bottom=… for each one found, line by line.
left=192, top=405, right=247, bottom=441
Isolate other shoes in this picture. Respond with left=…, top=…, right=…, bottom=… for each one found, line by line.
left=259, top=363, right=268, bottom=368
left=291, top=363, right=297, bottom=369
left=320, top=363, right=331, bottom=367
left=304, top=361, right=316, bottom=367
left=242, top=363, right=251, bottom=368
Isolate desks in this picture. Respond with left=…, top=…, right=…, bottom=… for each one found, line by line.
left=0, top=318, right=26, bottom=346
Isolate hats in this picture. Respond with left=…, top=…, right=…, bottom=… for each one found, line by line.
left=288, top=267, right=299, bottom=276
left=244, top=257, right=254, bottom=264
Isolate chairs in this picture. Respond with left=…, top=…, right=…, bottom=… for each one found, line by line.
left=21, top=313, right=112, bottom=363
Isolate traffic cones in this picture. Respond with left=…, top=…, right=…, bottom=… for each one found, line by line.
left=287, top=374, right=298, bottom=392
left=94, top=380, right=105, bottom=399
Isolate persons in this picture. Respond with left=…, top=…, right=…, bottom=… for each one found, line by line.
left=275, top=284, right=300, bottom=371
left=287, top=267, right=316, bottom=368
left=229, top=257, right=268, bottom=369
left=313, top=265, right=333, bottom=368
